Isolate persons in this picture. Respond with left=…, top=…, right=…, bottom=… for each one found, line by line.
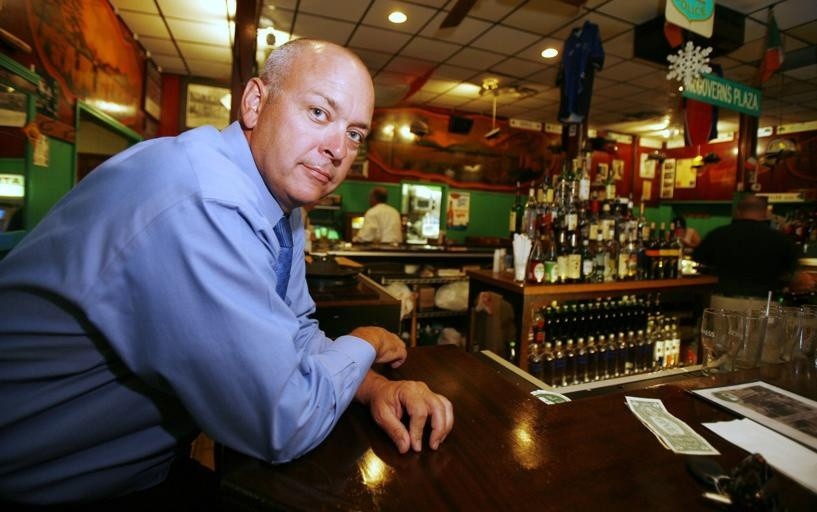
left=0, top=39, right=456, bottom=511
left=669, top=214, right=700, bottom=249
left=354, top=185, right=399, bottom=244
left=693, top=193, right=813, bottom=303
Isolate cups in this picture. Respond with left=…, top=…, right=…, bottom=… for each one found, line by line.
left=756, top=303, right=796, bottom=362
left=704, top=309, right=763, bottom=372
left=790, top=303, right=815, bottom=359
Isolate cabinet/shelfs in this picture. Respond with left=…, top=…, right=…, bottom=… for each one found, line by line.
left=356, top=259, right=501, bottom=348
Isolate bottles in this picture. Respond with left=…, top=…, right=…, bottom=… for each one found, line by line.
left=447, top=201, right=455, bottom=226
left=506, top=159, right=685, bottom=279
left=530, top=296, right=689, bottom=383
left=507, top=342, right=518, bottom=364
